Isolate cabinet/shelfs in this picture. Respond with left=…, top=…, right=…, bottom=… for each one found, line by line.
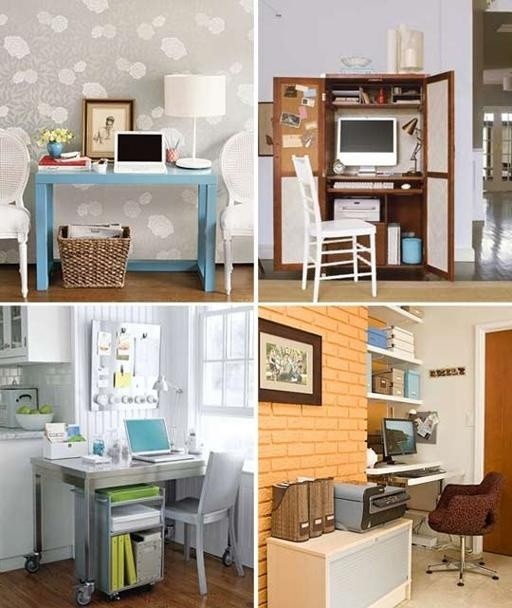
left=274, top=68, right=458, bottom=282
left=0, top=441, right=73, bottom=575
left=0, top=306, right=71, bottom=365
left=266, top=517, right=413, bottom=608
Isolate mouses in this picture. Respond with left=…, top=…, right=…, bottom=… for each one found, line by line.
left=401, top=184, right=411, bottom=190
left=437, top=469, right=446, bottom=474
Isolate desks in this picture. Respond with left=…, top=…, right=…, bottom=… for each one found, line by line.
left=33, top=158, right=218, bottom=293
left=366, top=458, right=448, bottom=548
left=22, top=452, right=237, bottom=605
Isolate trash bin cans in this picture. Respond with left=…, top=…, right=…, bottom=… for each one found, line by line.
left=402, top=238, right=422, bottom=264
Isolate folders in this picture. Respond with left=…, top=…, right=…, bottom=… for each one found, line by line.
left=111, top=534, right=137, bottom=592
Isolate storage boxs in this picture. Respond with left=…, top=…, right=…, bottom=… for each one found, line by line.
left=367, top=306, right=423, bottom=399
left=326, top=221, right=386, bottom=266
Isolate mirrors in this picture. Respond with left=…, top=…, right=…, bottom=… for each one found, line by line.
left=90, top=320, right=161, bottom=411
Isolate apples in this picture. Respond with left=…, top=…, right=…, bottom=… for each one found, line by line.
left=18, top=406, right=30, bottom=414
left=29, top=409, right=40, bottom=414
left=40, top=405, right=52, bottom=414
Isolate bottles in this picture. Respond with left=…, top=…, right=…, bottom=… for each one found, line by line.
left=187, top=433, right=198, bottom=453
left=92, top=433, right=132, bottom=467
left=376, top=87, right=386, bottom=105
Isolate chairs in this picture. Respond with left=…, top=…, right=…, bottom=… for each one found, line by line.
left=426, top=470, right=503, bottom=586
left=0, top=127, right=31, bottom=297
left=163, top=451, right=246, bottom=596
left=290, top=154, right=378, bottom=303
left=220, top=130, right=255, bottom=297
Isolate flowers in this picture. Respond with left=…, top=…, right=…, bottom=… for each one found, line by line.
left=34, top=126, right=76, bottom=145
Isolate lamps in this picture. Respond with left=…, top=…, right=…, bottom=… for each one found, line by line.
left=162, top=73, right=227, bottom=169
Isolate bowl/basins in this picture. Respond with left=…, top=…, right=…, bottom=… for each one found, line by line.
left=340, top=56, right=373, bottom=69
left=14, top=413, right=55, bottom=431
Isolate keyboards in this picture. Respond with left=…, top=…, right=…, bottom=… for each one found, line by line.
left=333, top=181, right=394, bottom=189
left=396, top=470, right=440, bottom=478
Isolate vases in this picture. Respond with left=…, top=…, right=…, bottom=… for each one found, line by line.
left=48, top=139, right=63, bottom=158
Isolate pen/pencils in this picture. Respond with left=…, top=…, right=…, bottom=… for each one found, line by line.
left=165, top=135, right=170, bottom=150
left=174, top=137, right=180, bottom=150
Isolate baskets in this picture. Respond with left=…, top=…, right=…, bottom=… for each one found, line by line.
left=55, top=224, right=133, bottom=291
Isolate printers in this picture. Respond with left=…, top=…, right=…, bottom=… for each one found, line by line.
left=333, top=480, right=410, bottom=530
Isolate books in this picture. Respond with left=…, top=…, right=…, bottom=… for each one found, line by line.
left=270, top=475, right=335, bottom=541
left=80, top=454, right=112, bottom=464
left=45, top=422, right=80, bottom=443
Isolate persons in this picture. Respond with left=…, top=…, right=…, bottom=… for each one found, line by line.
left=268, top=345, right=290, bottom=381
left=98, top=116, right=117, bottom=144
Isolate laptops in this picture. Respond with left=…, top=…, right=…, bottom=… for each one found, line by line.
left=114, top=131, right=167, bottom=174
left=124, top=417, right=195, bottom=463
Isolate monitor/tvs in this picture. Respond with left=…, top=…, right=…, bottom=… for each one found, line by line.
left=337, top=117, right=398, bottom=176
left=380, top=416, right=417, bottom=465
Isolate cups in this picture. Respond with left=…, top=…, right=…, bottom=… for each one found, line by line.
left=166, top=147, right=179, bottom=163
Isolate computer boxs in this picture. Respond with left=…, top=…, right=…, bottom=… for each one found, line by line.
left=388, top=222, right=402, bottom=266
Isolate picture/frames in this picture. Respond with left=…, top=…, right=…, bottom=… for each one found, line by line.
left=257, top=316, right=322, bottom=406
left=81, top=97, right=137, bottom=160
